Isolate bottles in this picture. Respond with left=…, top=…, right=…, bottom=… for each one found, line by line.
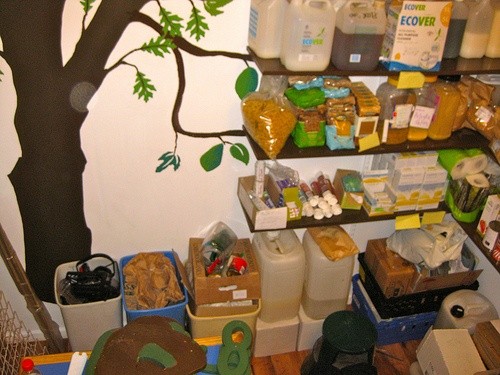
left=375, top=76, right=417, bottom=145
left=428, top=74, right=462, bottom=142
left=407, top=73, right=440, bottom=142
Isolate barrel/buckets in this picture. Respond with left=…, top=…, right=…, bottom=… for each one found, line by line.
left=443, top=0, right=500, bottom=59
left=251, top=229, right=306, bottom=322
left=248, top=0, right=388, bottom=72
left=435, top=288, right=500, bottom=335
left=302, top=230, right=355, bottom=321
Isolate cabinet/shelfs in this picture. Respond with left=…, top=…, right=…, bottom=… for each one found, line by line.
left=238, top=44, right=500, bottom=233
left=442, top=139, right=500, bottom=276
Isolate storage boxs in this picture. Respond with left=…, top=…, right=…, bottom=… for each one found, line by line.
left=19, top=151, right=500, bottom=374
left=382, top=0, right=454, bottom=71
